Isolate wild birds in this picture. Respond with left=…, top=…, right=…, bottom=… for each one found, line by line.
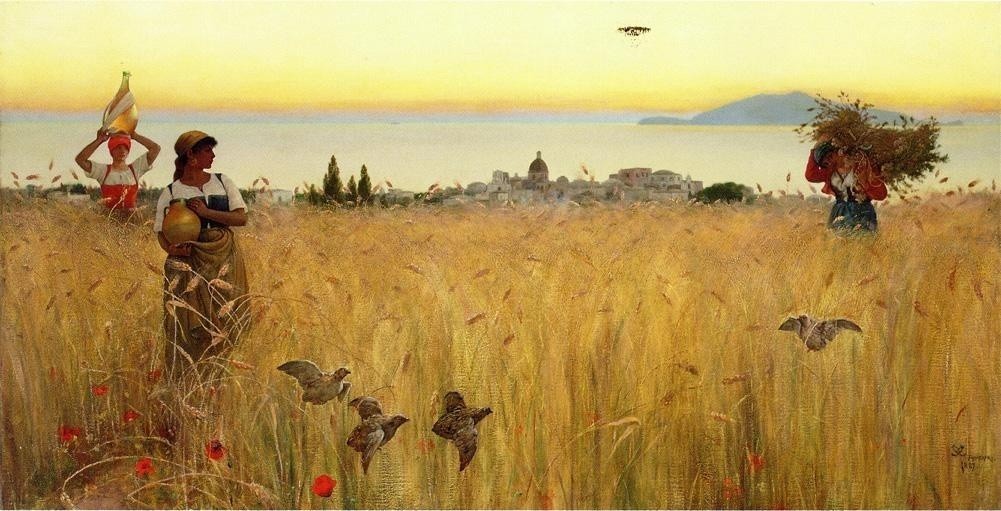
left=346, top=395, right=411, bottom=475
left=431, top=391, right=493, bottom=472
left=276, top=359, right=352, bottom=405
left=778, top=314, right=863, bottom=353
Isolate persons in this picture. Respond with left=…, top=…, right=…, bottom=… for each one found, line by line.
left=805, top=140, right=888, bottom=237
left=73, top=125, right=162, bottom=224
left=151, top=130, right=255, bottom=377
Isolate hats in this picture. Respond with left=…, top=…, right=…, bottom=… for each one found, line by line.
left=108, top=134, right=132, bottom=156
left=814, top=140, right=838, bottom=168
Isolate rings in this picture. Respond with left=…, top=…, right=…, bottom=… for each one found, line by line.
left=195, top=204, right=199, bottom=209
